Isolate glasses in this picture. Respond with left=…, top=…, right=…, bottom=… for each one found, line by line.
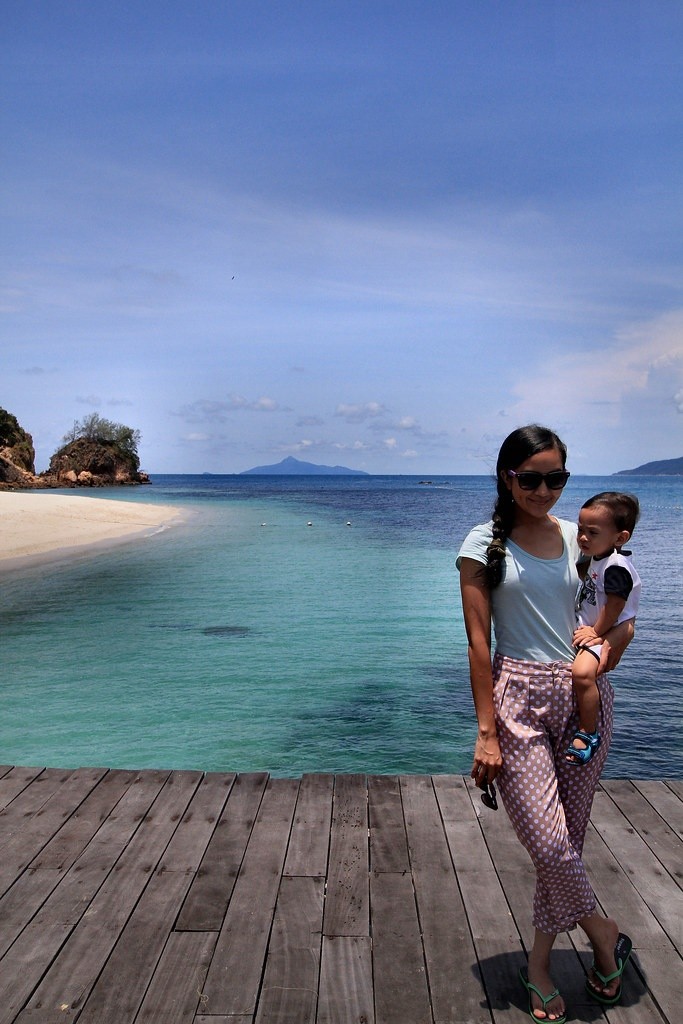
left=479, top=777, right=498, bottom=810
left=508, top=470, right=571, bottom=491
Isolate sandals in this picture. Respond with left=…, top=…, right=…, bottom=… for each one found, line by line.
left=563, top=729, right=602, bottom=766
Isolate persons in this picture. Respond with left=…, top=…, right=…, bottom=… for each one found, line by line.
left=564, top=491, right=640, bottom=765
left=455, top=425, right=637, bottom=1024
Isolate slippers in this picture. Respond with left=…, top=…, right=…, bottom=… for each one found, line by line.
left=584, top=932, right=633, bottom=1004
left=519, top=965, right=567, bottom=1024
